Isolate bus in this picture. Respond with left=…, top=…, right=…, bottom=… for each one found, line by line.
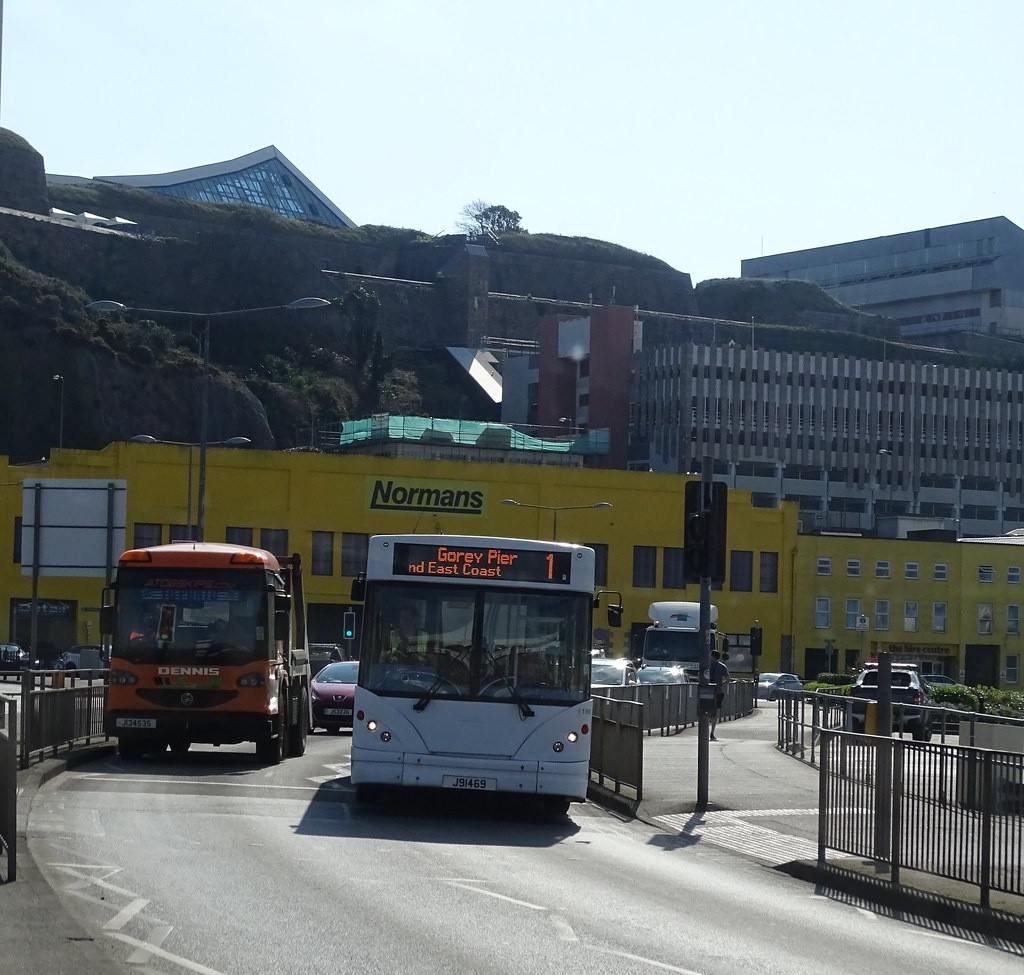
left=350, top=534, right=624, bottom=823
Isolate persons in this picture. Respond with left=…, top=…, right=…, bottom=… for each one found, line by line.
left=220, top=604, right=256, bottom=654
left=697, top=650, right=730, bottom=739
left=145, top=603, right=187, bottom=642
left=648, top=641, right=661, bottom=656
left=379, top=607, right=430, bottom=666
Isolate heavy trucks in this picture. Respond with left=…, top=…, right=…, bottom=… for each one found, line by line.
left=99, top=540, right=314, bottom=768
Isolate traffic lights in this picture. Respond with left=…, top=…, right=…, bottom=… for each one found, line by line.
left=156, top=605, right=176, bottom=643
left=343, top=612, right=355, bottom=639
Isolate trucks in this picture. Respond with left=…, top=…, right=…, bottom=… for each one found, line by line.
left=632, top=601, right=729, bottom=684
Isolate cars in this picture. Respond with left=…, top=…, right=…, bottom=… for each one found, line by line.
left=591, top=659, right=641, bottom=706
left=752, top=673, right=803, bottom=701
left=848, top=663, right=933, bottom=742
left=0, top=643, right=112, bottom=677
left=308, top=645, right=353, bottom=677
left=307, top=661, right=360, bottom=735
left=920, top=675, right=958, bottom=686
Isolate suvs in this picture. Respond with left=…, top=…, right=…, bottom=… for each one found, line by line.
left=636, top=664, right=691, bottom=699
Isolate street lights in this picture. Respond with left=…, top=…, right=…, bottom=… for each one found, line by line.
left=54, top=375, right=64, bottom=448
left=559, top=417, right=572, bottom=465
left=500, top=499, right=613, bottom=541
left=879, top=449, right=894, bottom=513
left=132, top=434, right=251, bottom=540
left=85, top=297, right=332, bottom=543
